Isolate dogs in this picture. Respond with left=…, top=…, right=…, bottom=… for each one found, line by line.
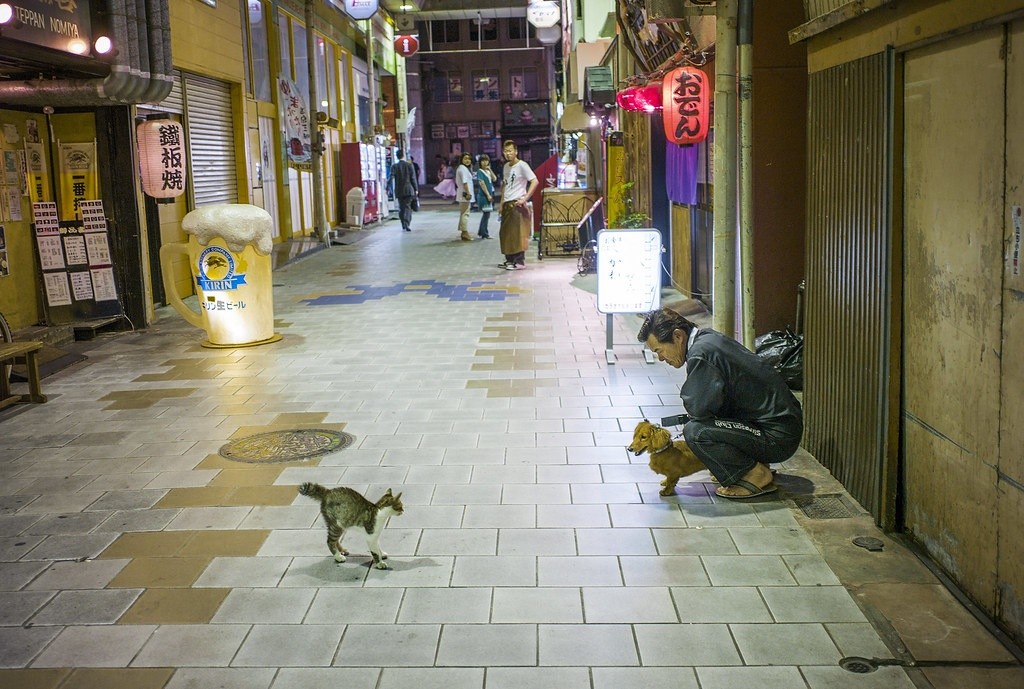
left=627, top=421, right=708, bottom=496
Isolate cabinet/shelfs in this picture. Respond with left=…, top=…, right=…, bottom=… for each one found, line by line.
left=541, top=186, right=596, bottom=257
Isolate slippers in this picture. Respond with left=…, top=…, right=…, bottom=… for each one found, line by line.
left=711, top=468, right=777, bottom=483
left=715, top=477, right=777, bottom=498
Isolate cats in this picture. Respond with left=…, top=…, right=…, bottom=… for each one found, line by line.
left=297, top=483, right=405, bottom=569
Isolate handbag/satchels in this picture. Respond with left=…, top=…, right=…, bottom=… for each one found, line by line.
left=413, top=196, right=420, bottom=211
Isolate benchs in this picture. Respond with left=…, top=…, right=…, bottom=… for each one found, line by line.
left=0, top=341, right=47, bottom=408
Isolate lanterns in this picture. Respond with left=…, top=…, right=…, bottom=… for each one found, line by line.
left=137, top=112, right=186, bottom=207
left=662, top=63, right=710, bottom=148
left=616, top=79, right=663, bottom=113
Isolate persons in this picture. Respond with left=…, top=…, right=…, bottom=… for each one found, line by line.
left=410, top=156, right=420, bottom=198
left=385, top=150, right=418, bottom=232
left=637, top=308, right=803, bottom=498
left=477, top=154, right=497, bottom=240
left=438, top=160, right=454, bottom=200
left=455, top=152, right=476, bottom=241
left=498, top=140, right=539, bottom=270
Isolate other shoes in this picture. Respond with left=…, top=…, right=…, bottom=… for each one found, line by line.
left=479, top=234, right=495, bottom=239
left=461, top=234, right=474, bottom=241
left=506, top=263, right=526, bottom=269
left=498, top=261, right=512, bottom=267
left=402, top=223, right=411, bottom=231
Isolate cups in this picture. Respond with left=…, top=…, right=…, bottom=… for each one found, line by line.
left=161, top=205, right=276, bottom=343
